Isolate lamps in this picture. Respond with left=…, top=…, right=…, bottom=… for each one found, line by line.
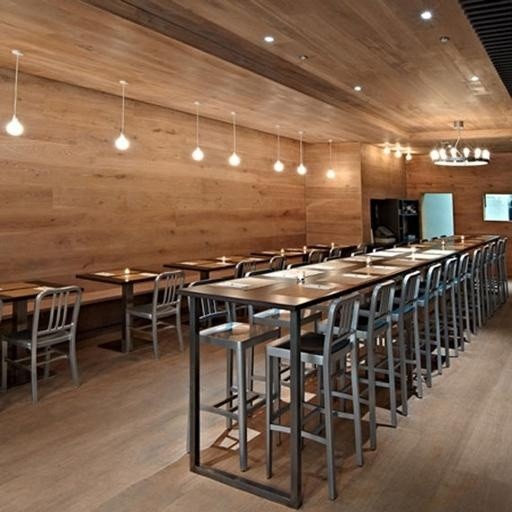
left=430, top=135, right=491, bottom=168
left=115, top=76, right=131, bottom=150
left=190, top=100, right=205, bottom=161
left=270, top=124, right=338, bottom=179
left=5, top=49, right=26, bottom=138
left=228, top=110, right=241, bottom=166
left=381, top=140, right=412, bottom=160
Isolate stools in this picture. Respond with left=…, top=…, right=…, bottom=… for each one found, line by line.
left=266, top=291, right=364, bottom=500
left=188, top=278, right=282, bottom=472
left=167, top=239, right=502, bottom=451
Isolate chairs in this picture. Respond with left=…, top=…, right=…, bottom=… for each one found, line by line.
left=124, top=268, right=185, bottom=359
left=4, top=285, right=82, bottom=402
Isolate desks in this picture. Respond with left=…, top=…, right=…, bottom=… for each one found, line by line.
left=4, top=280, right=84, bottom=387
left=77, top=267, right=165, bottom=347
left=176, top=236, right=497, bottom=510
